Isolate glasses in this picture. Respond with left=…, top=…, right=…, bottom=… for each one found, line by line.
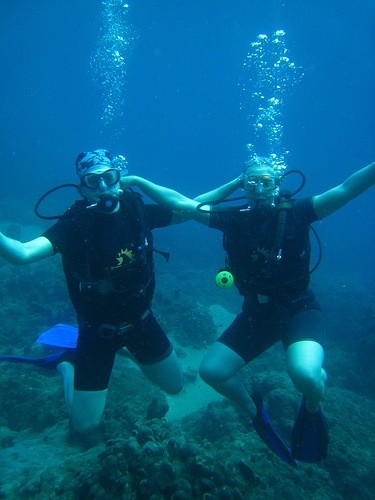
left=238, top=176, right=283, bottom=191
left=83, top=169, right=120, bottom=186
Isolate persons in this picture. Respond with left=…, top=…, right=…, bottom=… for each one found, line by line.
left=119, top=153, right=374, bottom=470
left=0, top=149, right=245, bottom=433
